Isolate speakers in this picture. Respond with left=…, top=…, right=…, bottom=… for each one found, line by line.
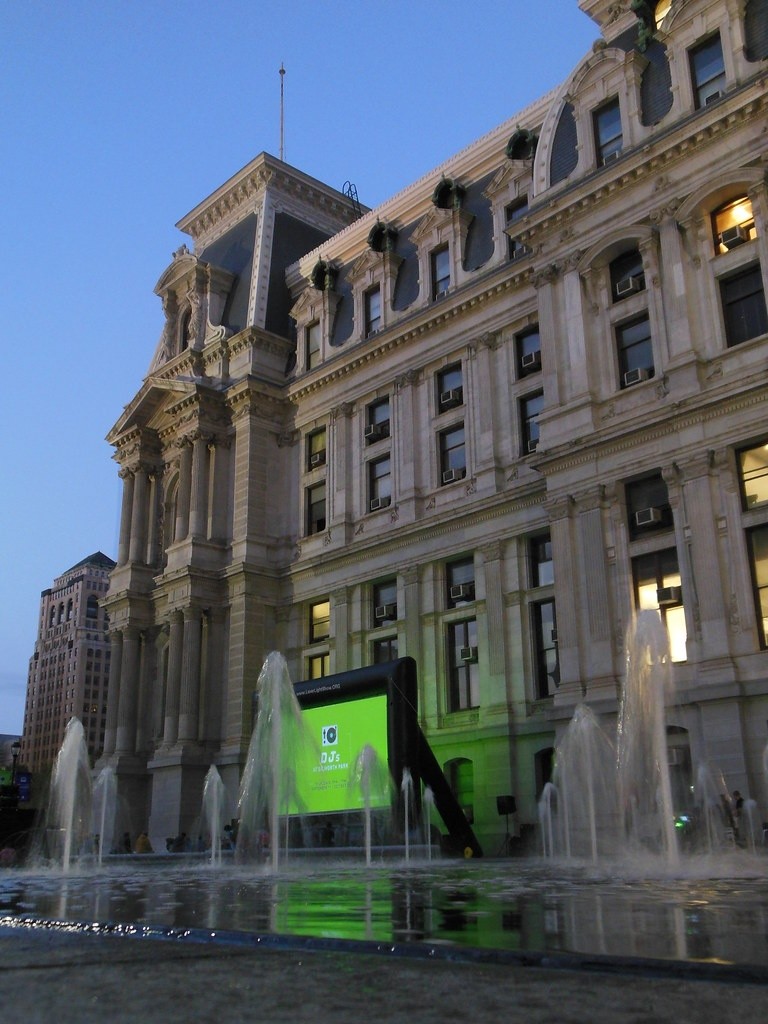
left=511, top=823, right=537, bottom=857
left=496, top=795, right=517, bottom=816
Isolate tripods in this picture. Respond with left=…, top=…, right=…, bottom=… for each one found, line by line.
left=497, top=814, right=513, bottom=859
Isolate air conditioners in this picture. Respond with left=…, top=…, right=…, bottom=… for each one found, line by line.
left=655, top=586, right=681, bottom=605
left=665, top=748, right=685, bottom=766
left=363, top=424, right=382, bottom=440
left=617, top=276, right=640, bottom=298
left=310, top=454, right=324, bottom=465
left=450, top=585, right=470, bottom=600
left=370, top=497, right=389, bottom=510
left=521, top=352, right=541, bottom=369
left=376, top=605, right=394, bottom=619
left=623, top=368, right=649, bottom=386
left=440, top=389, right=460, bottom=406
left=551, top=630, right=558, bottom=642
left=636, top=508, right=662, bottom=526
left=460, top=647, right=477, bottom=661
left=442, top=468, right=462, bottom=484
left=721, top=225, right=747, bottom=249
left=527, top=437, right=539, bottom=453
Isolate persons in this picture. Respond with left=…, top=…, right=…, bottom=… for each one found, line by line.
left=186, top=287, right=202, bottom=339
left=90, top=814, right=439, bottom=854
left=677, top=791, right=748, bottom=847
left=160, top=308, right=175, bottom=357
